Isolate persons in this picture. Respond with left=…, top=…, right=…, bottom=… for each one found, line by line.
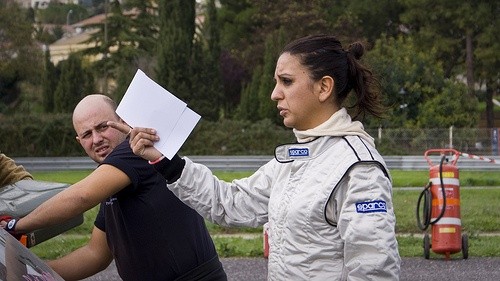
left=107, top=33, right=401, bottom=281
left=0, top=153, right=34, bottom=187
left=0, top=94, right=227, bottom=281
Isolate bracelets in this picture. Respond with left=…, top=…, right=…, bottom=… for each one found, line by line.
left=5, top=218, right=20, bottom=233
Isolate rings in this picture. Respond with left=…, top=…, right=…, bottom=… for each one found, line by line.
left=127, top=127, right=131, bottom=136
